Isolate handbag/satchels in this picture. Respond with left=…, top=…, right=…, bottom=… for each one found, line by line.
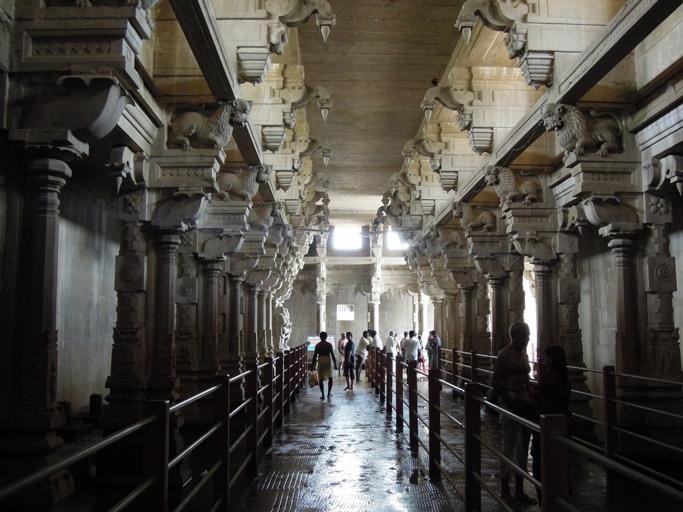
left=307, top=371, right=319, bottom=388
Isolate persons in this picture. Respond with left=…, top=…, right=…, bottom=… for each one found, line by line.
left=310, top=331, right=337, bottom=399
left=492, top=321, right=571, bottom=508
left=339, top=330, right=441, bottom=391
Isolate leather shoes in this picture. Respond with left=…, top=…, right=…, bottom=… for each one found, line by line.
left=320, top=395, right=330, bottom=400
